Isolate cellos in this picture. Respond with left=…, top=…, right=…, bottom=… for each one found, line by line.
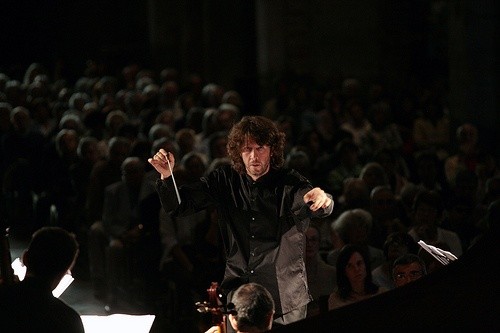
left=195, top=282, right=237, bottom=333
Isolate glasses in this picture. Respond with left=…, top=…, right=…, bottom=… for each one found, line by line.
left=416, top=206, right=439, bottom=215
left=304, top=236, right=320, bottom=244
left=394, top=271, right=423, bottom=284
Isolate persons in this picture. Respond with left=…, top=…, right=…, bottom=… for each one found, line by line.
left=0, top=226, right=86, bottom=333
left=0, top=52, right=500, bottom=309
left=228, top=283, right=276, bottom=333
left=148, top=116, right=334, bottom=333
